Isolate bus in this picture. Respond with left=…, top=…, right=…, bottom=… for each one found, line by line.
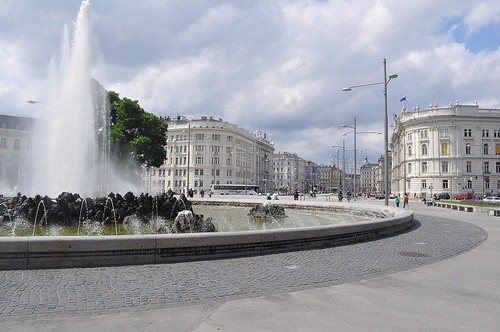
left=211, top=184, right=260, bottom=196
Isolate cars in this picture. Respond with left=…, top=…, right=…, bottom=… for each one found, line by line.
left=453, top=193, right=475, bottom=200
left=435, top=193, right=450, bottom=200
left=482, top=197, right=500, bottom=202
left=356, top=191, right=397, bottom=199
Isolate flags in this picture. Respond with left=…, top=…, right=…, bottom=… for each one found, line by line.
left=400, top=96, right=406, bottom=102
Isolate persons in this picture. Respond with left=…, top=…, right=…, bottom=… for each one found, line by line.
left=200, top=189, right=204, bottom=197
left=189, top=188, right=195, bottom=197
left=345, top=191, right=351, bottom=202
left=167, top=188, right=173, bottom=197
left=429, top=197, right=435, bottom=205
left=476, top=195, right=483, bottom=200
left=338, top=189, right=343, bottom=201
left=403, top=193, right=409, bottom=208
left=293, top=188, right=300, bottom=200
left=208, top=188, right=213, bottom=197
left=272, top=191, right=279, bottom=200
left=395, top=196, right=400, bottom=207
left=266, top=191, right=271, bottom=200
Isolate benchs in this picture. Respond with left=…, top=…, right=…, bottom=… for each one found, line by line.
left=425, top=201, right=500, bottom=216
left=299, top=193, right=333, bottom=203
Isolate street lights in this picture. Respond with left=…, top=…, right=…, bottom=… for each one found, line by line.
left=26, top=100, right=56, bottom=144
left=342, top=58, right=399, bottom=206
left=467, top=137, right=492, bottom=199
left=342, top=117, right=381, bottom=200
left=329, top=140, right=363, bottom=195
left=182, top=122, right=211, bottom=199
